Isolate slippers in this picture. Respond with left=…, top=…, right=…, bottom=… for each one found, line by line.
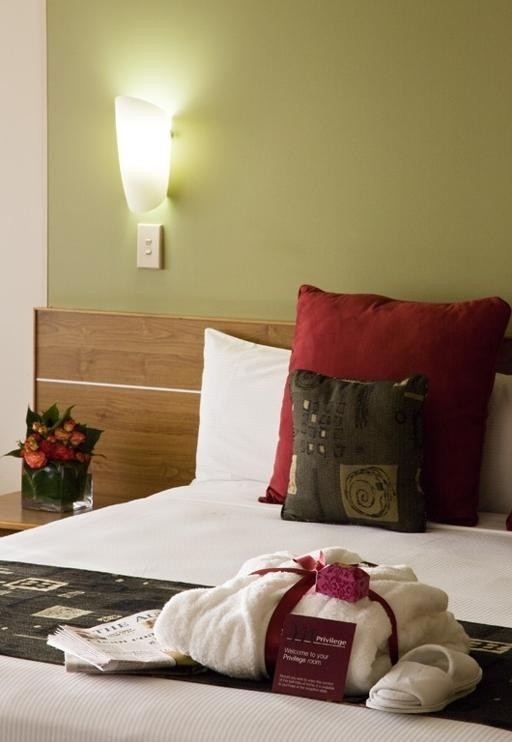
left=362, top=641, right=488, bottom=714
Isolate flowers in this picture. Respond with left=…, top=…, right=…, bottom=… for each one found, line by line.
left=2, top=397, right=107, bottom=508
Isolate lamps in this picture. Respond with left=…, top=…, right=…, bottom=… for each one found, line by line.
left=115, top=94, right=169, bottom=212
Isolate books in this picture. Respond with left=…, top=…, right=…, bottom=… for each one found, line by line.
left=270, top=611, right=358, bottom=704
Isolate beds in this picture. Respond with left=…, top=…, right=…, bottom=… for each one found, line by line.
left=1, top=308, right=511, bottom=742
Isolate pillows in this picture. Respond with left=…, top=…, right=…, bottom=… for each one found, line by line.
left=280, top=367, right=429, bottom=534
left=477, top=371, right=512, bottom=515
left=259, top=285, right=510, bottom=526
left=188, top=326, right=292, bottom=481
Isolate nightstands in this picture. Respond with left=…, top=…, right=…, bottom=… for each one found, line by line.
left=0, top=491, right=134, bottom=537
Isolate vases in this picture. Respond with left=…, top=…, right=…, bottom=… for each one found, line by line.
left=20, top=460, right=93, bottom=513
